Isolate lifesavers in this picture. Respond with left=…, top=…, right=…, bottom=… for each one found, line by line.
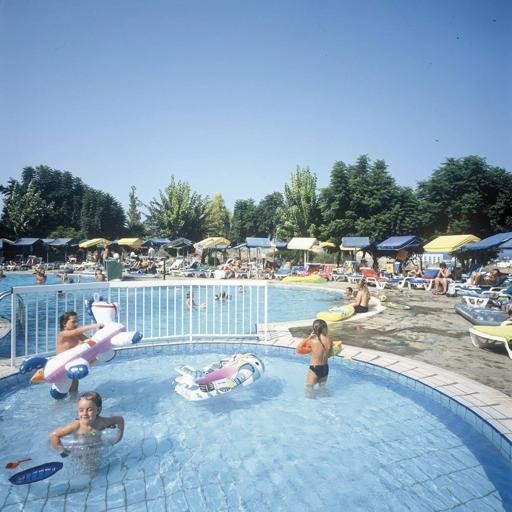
left=174, top=353, right=265, bottom=401
left=214, top=294, right=233, bottom=303
left=20, top=293, right=142, bottom=399
left=297, top=339, right=341, bottom=357
left=50, top=424, right=122, bottom=451
left=317, top=305, right=355, bottom=321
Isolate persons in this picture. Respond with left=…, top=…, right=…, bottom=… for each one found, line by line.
left=0, top=244, right=274, bottom=312
left=431, top=261, right=455, bottom=295
left=50, top=391, right=125, bottom=480
left=352, top=279, right=370, bottom=313
left=56, top=310, right=106, bottom=401
left=408, top=264, right=420, bottom=278
left=315, top=286, right=353, bottom=304
left=297, top=318, right=333, bottom=399
left=467, top=267, right=500, bottom=289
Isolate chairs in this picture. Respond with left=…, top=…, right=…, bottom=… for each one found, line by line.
left=156, top=258, right=445, bottom=294
left=445, top=259, right=512, bottom=359
